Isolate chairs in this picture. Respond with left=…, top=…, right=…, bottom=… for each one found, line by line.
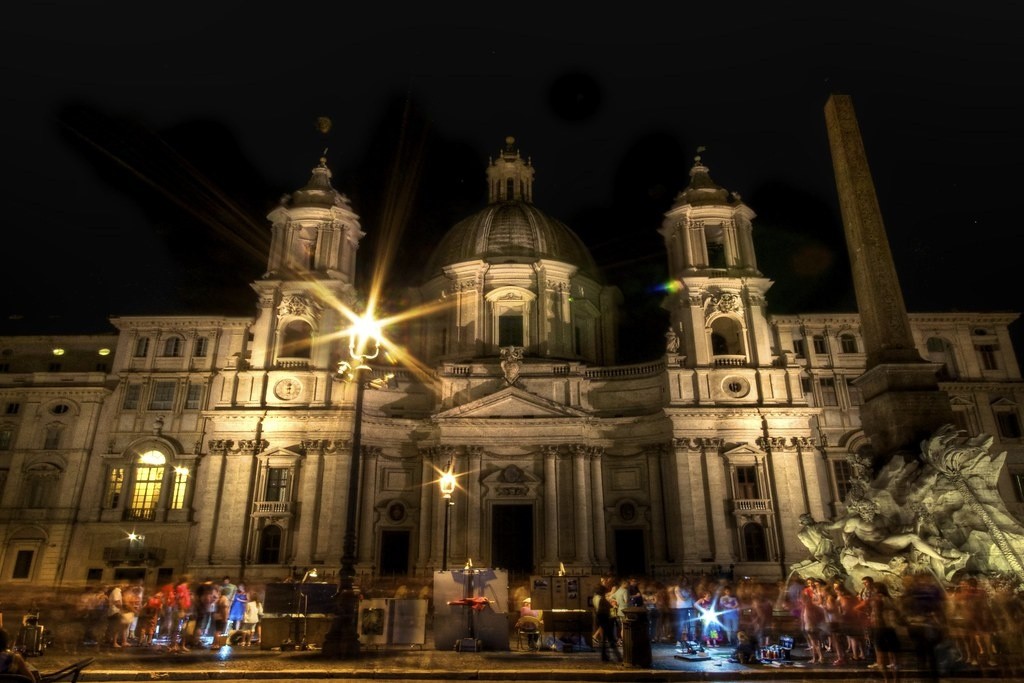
left=518, top=616, right=545, bottom=653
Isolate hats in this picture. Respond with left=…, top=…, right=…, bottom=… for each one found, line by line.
left=523, top=598, right=531, bottom=603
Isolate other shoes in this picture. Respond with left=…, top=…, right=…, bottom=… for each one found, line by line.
left=592, top=637, right=598, bottom=643
left=707, top=643, right=720, bottom=647
left=833, top=660, right=845, bottom=666
left=886, top=664, right=898, bottom=669
left=804, top=646, right=813, bottom=651
left=956, top=657, right=996, bottom=666
left=238, top=642, right=251, bottom=647
left=137, top=643, right=142, bottom=647
left=868, top=663, right=882, bottom=670
left=850, top=656, right=866, bottom=661
left=146, top=643, right=151, bottom=647
left=807, top=659, right=826, bottom=664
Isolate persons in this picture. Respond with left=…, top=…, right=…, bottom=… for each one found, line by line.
left=797, top=454, right=873, bottom=573
left=842, top=499, right=963, bottom=568
left=519, top=597, right=543, bottom=652
left=591, top=573, right=1024, bottom=683
left=0, top=576, right=263, bottom=683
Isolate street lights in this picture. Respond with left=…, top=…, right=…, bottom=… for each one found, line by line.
left=321, top=315, right=383, bottom=663
left=440, top=473, right=457, bottom=571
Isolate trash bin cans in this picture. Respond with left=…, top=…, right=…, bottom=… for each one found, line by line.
left=620, top=606, right=654, bottom=669
